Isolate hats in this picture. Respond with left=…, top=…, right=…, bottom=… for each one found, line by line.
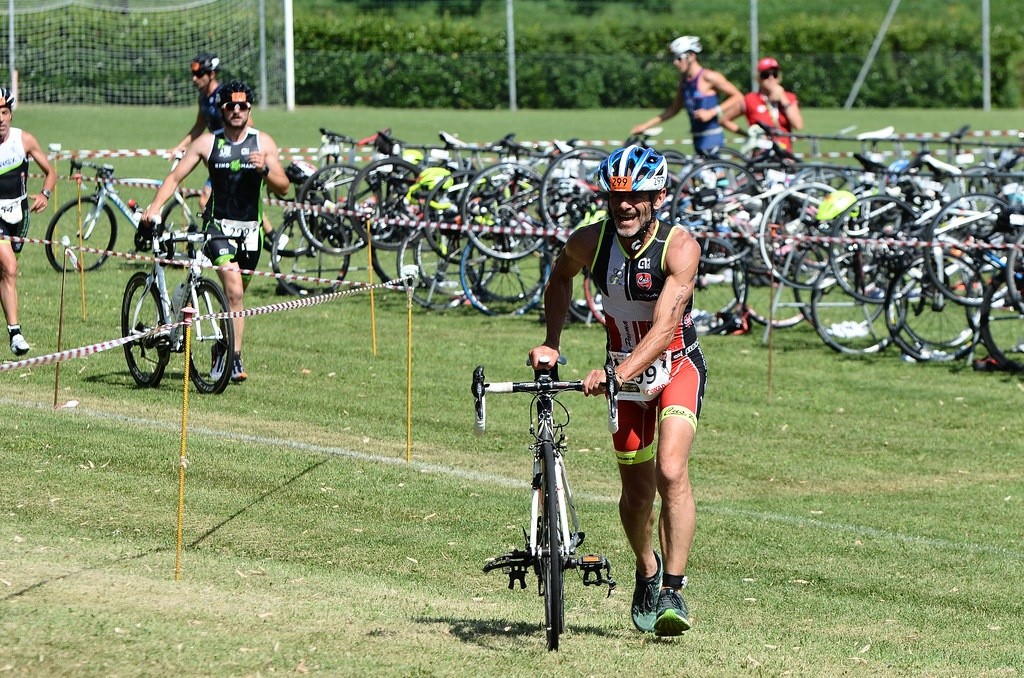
left=758, top=57, right=779, bottom=70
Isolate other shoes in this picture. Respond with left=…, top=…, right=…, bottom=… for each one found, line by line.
left=9, top=333, right=30, bottom=356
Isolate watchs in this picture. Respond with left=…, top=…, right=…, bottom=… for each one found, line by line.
left=42, top=190, right=50, bottom=199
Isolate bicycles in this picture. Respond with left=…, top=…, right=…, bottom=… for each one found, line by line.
left=263, top=124, right=1024, bottom=379
left=121, top=221, right=250, bottom=396
left=470, top=350, right=619, bottom=653
left=44, top=156, right=206, bottom=273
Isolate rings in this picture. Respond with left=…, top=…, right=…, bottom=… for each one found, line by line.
left=596, top=382, right=599, bottom=383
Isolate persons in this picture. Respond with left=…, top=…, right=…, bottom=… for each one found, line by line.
left=718, top=57, right=803, bottom=154
left=529, top=143, right=708, bottom=638
left=0, top=85, right=55, bottom=357
left=140, top=81, right=288, bottom=382
left=630, top=36, right=744, bottom=286
left=165, top=53, right=289, bottom=267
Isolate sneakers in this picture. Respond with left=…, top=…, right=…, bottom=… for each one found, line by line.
left=210, top=345, right=224, bottom=380
left=631, top=549, right=663, bottom=632
left=653, top=588, right=692, bottom=636
left=231, top=356, right=248, bottom=381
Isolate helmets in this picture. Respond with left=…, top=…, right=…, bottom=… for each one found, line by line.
left=0, top=87, right=16, bottom=111
left=597, top=144, right=668, bottom=192
left=402, top=149, right=424, bottom=164
left=669, top=36, right=703, bottom=55
left=215, top=80, right=256, bottom=108
left=418, top=167, right=454, bottom=190
left=816, top=190, right=857, bottom=220
left=191, top=51, right=220, bottom=72
left=286, top=161, right=318, bottom=184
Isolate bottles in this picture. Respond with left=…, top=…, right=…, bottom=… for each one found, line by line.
left=128, top=197, right=144, bottom=215
left=172, top=282, right=184, bottom=313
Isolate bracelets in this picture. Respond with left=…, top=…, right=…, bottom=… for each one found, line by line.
left=781, top=103, right=791, bottom=110
left=616, top=371, right=625, bottom=386
left=715, top=105, right=721, bottom=116
left=259, top=166, right=269, bottom=177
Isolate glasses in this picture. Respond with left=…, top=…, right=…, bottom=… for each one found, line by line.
left=761, top=70, right=779, bottom=79
left=675, top=56, right=688, bottom=60
left=222, top=102, right=252, bottom=111
left=193, top=69, right=209, bottom=78
